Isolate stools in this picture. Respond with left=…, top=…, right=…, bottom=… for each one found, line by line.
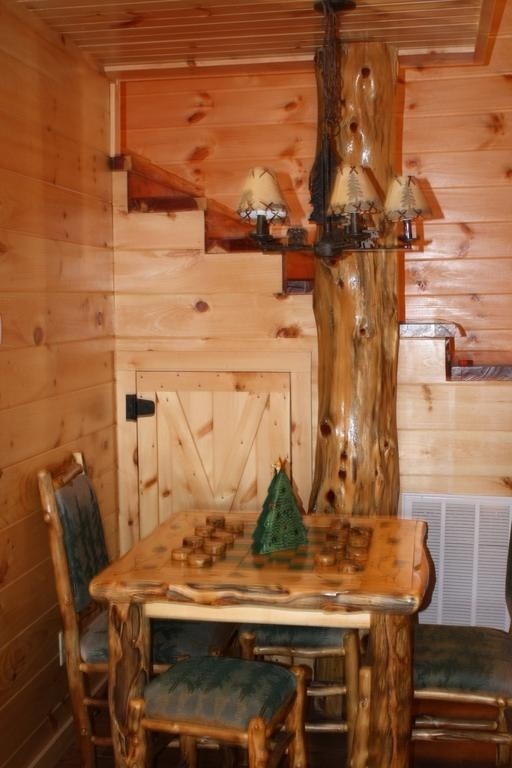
left=129, top=649, right=313, bottom=768
left=239, top=626, right=357, bottom=768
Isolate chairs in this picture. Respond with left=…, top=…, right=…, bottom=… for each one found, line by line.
left=350, top=502, right=511, bottom=768
left=35, top=450, right=227, bottom=768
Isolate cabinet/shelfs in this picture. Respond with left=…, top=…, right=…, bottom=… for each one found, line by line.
left=121, top=362, right=293, bottom=547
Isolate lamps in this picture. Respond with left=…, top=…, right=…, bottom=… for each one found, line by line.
left=236, top=0, right=432, bottom=264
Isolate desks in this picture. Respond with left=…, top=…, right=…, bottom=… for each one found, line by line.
left=88, top=505, right=435, bottom=763
